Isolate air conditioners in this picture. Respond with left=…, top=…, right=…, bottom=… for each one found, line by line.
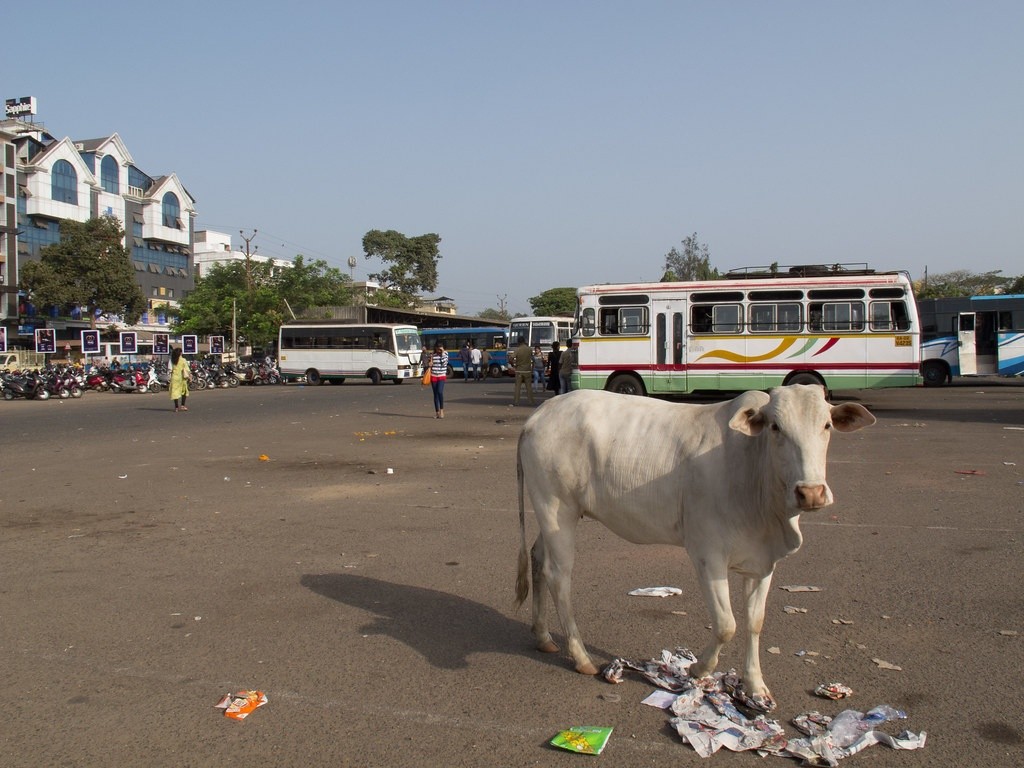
left=75, top=143, right=84, bottom=151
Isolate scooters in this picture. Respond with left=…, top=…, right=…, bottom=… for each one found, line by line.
left=0, top=356, right=308, bottom=400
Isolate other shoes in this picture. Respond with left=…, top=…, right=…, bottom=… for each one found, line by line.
left=181, top=406, right=189, bottom=411
left=175, top=408, right=180, bottom=412
left=440, top=409, right=445, bottom=419
left=434, top=412, right=439, bottom=419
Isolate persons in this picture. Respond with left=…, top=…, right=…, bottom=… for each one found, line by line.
left=418, top=346, right=431, bottom=375
left=458, top=342, right=493, bottom=382
left=47, top=357, right=121, bottom=371
left=495, top=336, right=573, bottom=406
left=430, top=342, right=449, bottom=418
left=168, top=347, right=191, bottom=412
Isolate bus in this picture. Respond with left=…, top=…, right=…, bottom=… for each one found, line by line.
left=567, top=261, right=924, bottom=404
left=505, top=316, right=608, bottom=384
left=406, top=326, right=519, bottom=380
left=920, top=294, right=1024, bottom=388
left=277, top=323, right=428, bottom=387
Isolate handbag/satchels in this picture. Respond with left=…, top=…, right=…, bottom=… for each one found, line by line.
left=421, top=354, right=433, bottom=385
left=182, top=366, right=190, bottom=380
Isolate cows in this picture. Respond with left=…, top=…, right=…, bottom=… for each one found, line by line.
left=513, top=383, right=877, bottom=713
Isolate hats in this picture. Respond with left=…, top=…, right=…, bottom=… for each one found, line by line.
left=551, top=342, right=560, bottom=347
